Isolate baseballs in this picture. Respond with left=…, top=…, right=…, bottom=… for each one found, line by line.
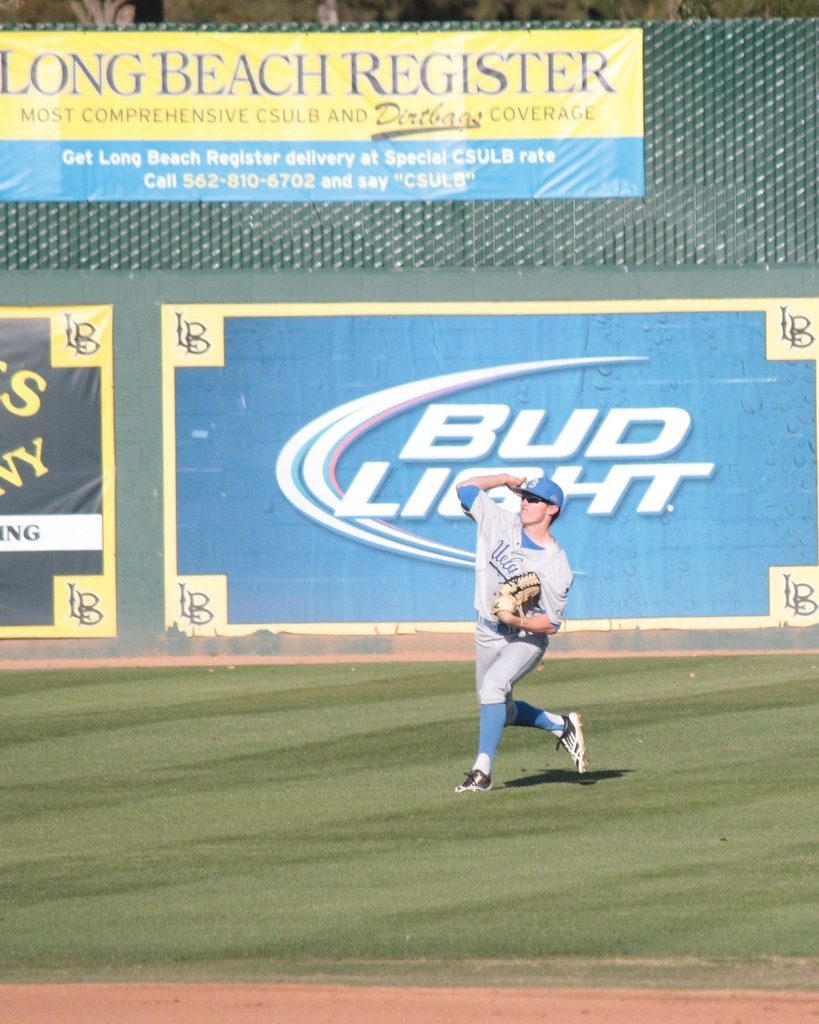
left=521, top=481, right=529, bottom=488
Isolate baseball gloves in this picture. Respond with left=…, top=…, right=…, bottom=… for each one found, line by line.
left=490, top=571, right=542, bottom=615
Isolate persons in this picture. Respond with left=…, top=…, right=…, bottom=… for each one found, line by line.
left=454, top=473, right=587, bottom=793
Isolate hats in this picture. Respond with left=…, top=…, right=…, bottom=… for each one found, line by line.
left=513, top=478, right=563, bottom=507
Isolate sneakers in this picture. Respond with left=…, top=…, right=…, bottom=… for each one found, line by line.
left=454, top=769, right=492, bottom=793
left=555, top=712, right=587, bottom=774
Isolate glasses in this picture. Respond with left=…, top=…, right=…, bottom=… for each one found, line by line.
left=521, top=491, right=550, bottom=503
